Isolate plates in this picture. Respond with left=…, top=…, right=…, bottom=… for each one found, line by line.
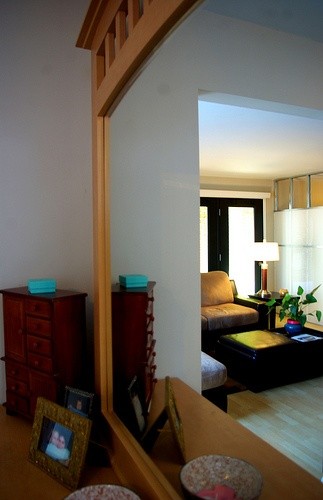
left=180, top=455, right=263, bottom=500
left=65, top=484, right=140, bottom=500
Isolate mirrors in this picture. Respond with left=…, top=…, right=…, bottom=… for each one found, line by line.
left=73, top=0, right=323, bottom=500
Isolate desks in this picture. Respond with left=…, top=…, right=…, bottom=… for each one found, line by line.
left=215, top=327, right=323, bottom=394
left=149, top=377, right=323, bottom=500
left=0, top=406, right=122, bottom=500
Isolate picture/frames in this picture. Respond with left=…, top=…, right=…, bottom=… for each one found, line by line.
left=25, top=396, right=93, bottom=492
left=125, top=375, right=148, bottom=442
left=62, top=384, right=95, bottom=419
left=140, top=377, right=188, bottom=466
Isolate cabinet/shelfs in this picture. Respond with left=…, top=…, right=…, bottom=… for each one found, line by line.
left=0, top=286, right=88, bottom=425
left=111, top=281, right=158, bottom=423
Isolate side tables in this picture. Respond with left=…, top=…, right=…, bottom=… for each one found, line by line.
left=248, top=291, right=300, bottom=329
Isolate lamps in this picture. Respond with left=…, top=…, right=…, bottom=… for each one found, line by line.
left=252, top=242, right=280, bottom=295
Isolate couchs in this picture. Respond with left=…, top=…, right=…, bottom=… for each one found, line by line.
left=201, top=271, right=265, bottom=354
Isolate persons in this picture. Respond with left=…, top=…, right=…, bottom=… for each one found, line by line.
left=45, top=429, right=73, bottom=467
left=67, top=399, right=87, bottom=418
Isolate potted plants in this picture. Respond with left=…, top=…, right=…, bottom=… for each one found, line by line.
left=265, top=283, right=323, bottom=336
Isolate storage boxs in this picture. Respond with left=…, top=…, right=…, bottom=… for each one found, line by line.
left=27, top=278, right=57, bottom=294
left=119, top=273, right=150, bottom=288
left=117, top=404, right=147, bottom=433
